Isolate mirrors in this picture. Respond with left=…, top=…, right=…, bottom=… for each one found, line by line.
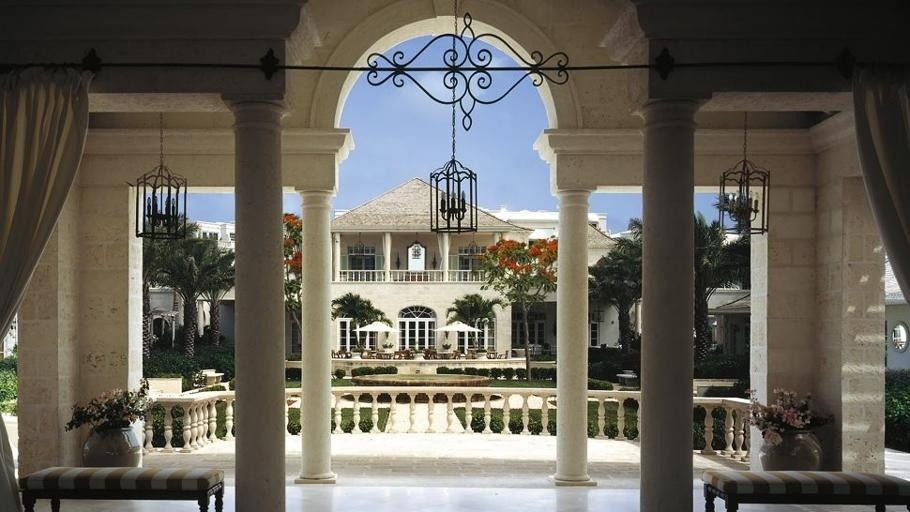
left=889, top=320, right=910, bottom=353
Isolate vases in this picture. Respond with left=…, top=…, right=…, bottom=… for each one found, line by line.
left=757, top=429, right=823, bottom=471
left=81, top=424, right=144, bottom=468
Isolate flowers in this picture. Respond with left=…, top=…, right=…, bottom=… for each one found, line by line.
left=745, top=386, right=826, bottom=444
left=57, top=373, right=155, bottom=429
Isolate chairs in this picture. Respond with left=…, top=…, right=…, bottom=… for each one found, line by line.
left=513, top=342, right=553, bottom=357
left=332, top=347, right=511, bottom=361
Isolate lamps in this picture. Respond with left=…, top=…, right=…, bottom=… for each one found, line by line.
left=135, top=110, right=186, bottom=243
left=715, top=111, right=773, bottom=235
left=430, top=0, right=479, bottom=235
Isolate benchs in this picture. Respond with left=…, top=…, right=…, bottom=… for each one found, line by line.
left=19, top=465, right=227, bottom=512
left=701, top=465, right=909, bottom=511
